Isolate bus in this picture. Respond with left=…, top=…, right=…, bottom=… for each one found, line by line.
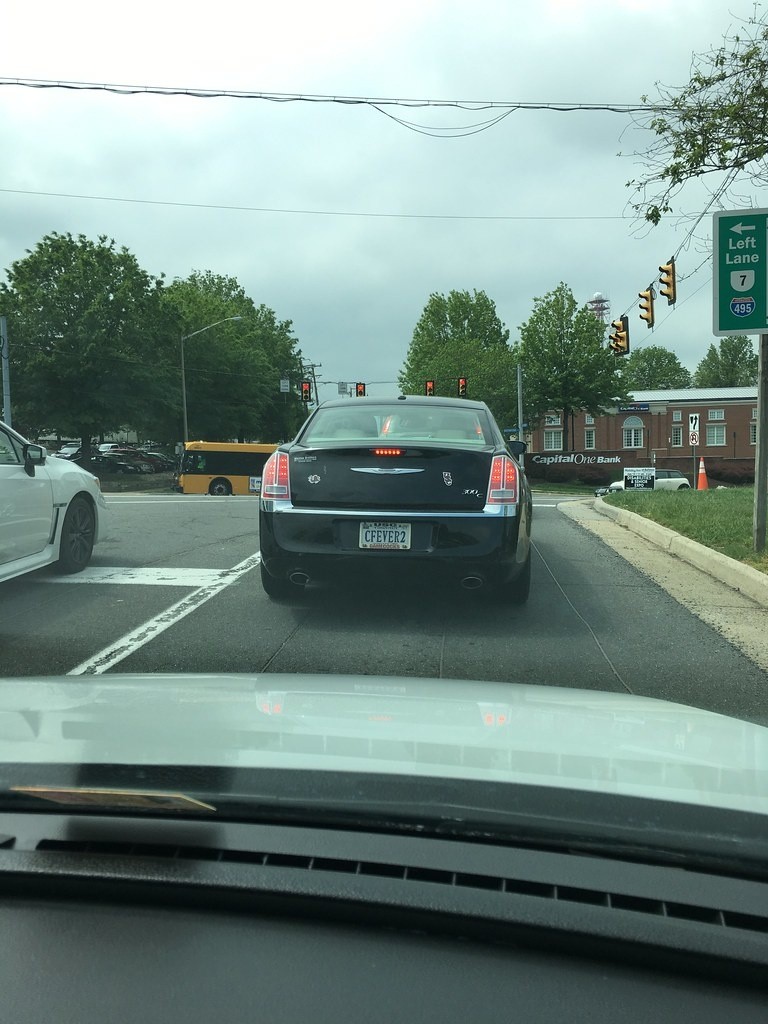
left=175, top=441, right=280, bottom=496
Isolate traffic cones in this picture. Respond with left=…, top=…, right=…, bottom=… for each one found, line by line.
left=696, top=456, right=710, bottom=491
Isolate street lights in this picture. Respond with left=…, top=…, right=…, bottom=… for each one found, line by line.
left=180, top=316, right=242, bottom=442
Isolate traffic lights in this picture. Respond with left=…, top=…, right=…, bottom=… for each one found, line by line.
left=657, top=258, right=677, bottom=307
left=355, top=383, right=365, bottom=397
left=458, top=376, right=467, bottom=397
left=608, top=315, right=630, bottom=357
left=426, top=380, right=435, bottom=396
left=638, top=285, right=654, bottom=329
left=301, top=382, right=311, bottom=403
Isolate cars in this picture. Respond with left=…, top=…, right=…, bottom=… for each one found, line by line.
left=258, top=394, right=534, bottom=608
left=608, top=469, right=692, bottom=494
left=1, top=421, right=113, bottom=583
left=51, top=439, right=209, bottom=475
